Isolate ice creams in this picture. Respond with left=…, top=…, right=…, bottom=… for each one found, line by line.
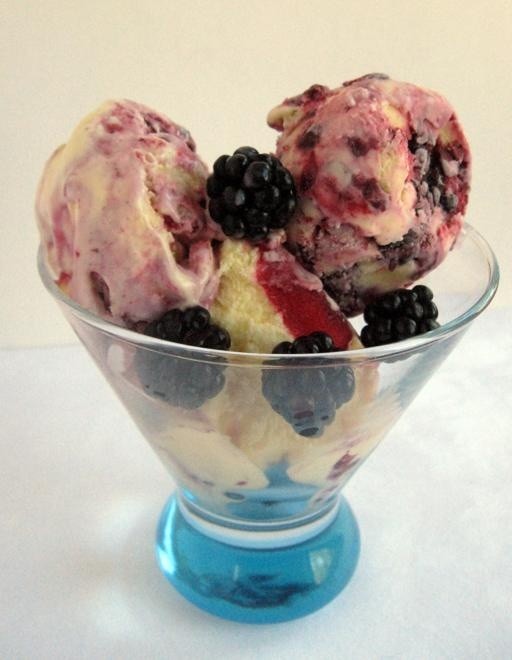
left=33, top=72, right=472, bottom=507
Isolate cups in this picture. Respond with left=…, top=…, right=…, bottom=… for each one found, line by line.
left=35, top=221, right=500, bottom=625
